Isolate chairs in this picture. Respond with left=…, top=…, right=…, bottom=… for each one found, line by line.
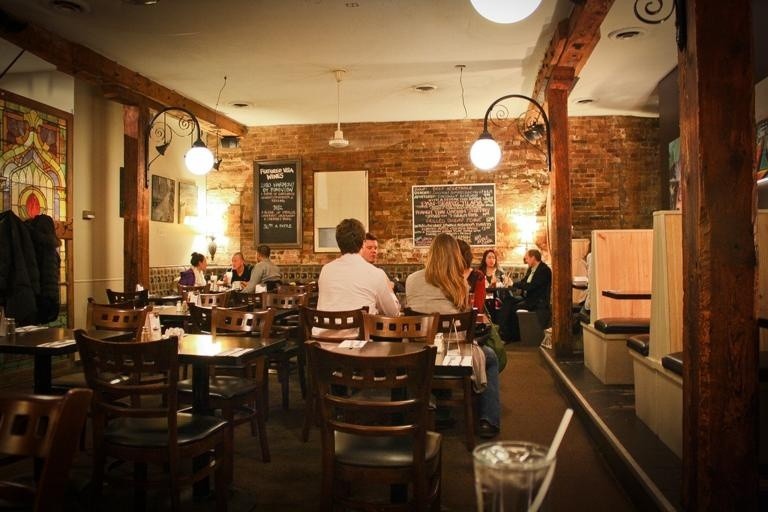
left=594, top=227, right=655, bottom=385
left=658, top=211, right=768, bottom=459
left=32, top=297, right=148, bottom=453
left=176, top=282, right=211, bottom=296
left=404, top=307, right=477, bottom=451
left=0, top=388, right=93, bottom=512
left=297, top=303, right=369, bottom=444
left=185, top=292, right=226, bottom=307
left=278, top=286, right=313, bottom=307
left=580, top=230, right=595, bottom=376
left=625, top=211, right=658, bottom=416
left=515, top=264, right=553, bottom=347
left=73, top=329, right=230, bottom=512
left=232, top=290, right=265, bottom=307
left=256, top=292, right=308, bottom=412
left=342, top=311, right=439, bottom=434
left=304, top=340, right=443, bottom=512
left=87, top=297, right=132, bottom=310
left=176, top=305, right=276, bottom=463
left=188, top=301, right=256, bottom=418
left=107, top=289, right=149, bottom=304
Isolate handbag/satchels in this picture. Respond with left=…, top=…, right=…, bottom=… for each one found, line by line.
left=483, top=303, right=507, bottom=373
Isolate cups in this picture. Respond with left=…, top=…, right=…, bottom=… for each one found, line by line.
left=473, top=440, right=558, bottom=512
left=396, top=292, right=407, bottom=312
left=210, top=275, right=217, bottom=290
left=226, top=271, right=232, bottom=286
left=469, top=293, right=476, bottom=307
left=503, top=276, right=509, bottom=288
left=490, top=273, right=496, bottom=288
left=234, top=281, right=241, bottom=290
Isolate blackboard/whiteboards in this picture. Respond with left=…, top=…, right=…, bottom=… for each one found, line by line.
left=412, top=182, right=497, bottom=249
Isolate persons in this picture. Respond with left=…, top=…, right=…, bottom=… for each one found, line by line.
left=502, top=249, right=551, bottom=344
left=177, top=252, right=206, bottom=288
left=362, top=233, right=398, bottom=294
left=405, top=233, right=501, bottom=436
left=219, top=252, right=254, bottom=282
left=240, top=245, right=280, bottom=294
left=311, top=218, right=404, bottom=426
left=479, top=249, right=513, bottom=299
left=456, top=238, right=486, bottom=313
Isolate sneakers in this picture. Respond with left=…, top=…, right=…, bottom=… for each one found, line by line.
left=435, top=416, right=458, bottom=430
left=478, top=420, right=500, bottom=439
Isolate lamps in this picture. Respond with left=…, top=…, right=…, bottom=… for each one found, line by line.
left=329, top=69, right=350, bottom=148
left=145, top=105, right=214, bottom=191
left=471, top=95, right=551, bottom=173
